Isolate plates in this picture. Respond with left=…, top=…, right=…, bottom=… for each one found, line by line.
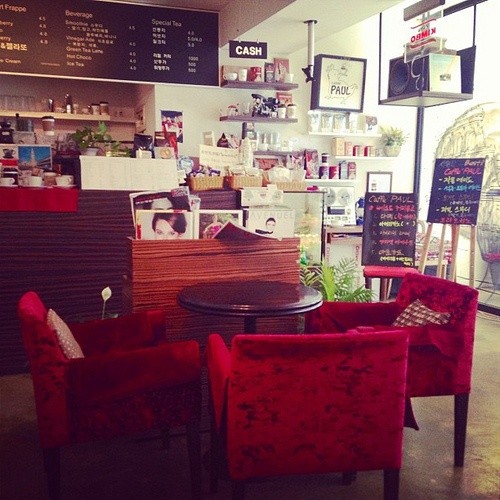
left=22, top=185, right=46, bottom=188
left=53, top=185, right=77, bottom=188
left=0, top=185, right=18, bottom=187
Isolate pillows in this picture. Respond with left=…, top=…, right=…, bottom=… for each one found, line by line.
left=390, top=299, right=453, bottom=327
left=46, top=308, right=85, bottom=359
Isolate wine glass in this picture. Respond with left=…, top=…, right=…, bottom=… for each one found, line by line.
left=241, top=103, right=251, bottom=118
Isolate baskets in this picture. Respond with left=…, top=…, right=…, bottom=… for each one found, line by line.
left=226, top=175, right=262, bottom=189
left=186, top=177, right=223, bottom=191
left=277, top=182, right=307, bottom=191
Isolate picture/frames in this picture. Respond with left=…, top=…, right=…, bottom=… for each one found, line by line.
left=366, top=171, right=393, bottom=192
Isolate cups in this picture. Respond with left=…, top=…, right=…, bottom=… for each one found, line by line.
left=278, top=105, right=287, bottom=119
left=259, top=144, right=269, bottom=152
left=55, top=175, right=74, bottom=185
left=223, top=73, right=238, bottom=81
left=227, top=108, right=236, bottom=117
left=29, top=176, right=42, bottom=186
left=282, top=141, right=291, bottom=147
left=73, top=102, right=108, bottom=115
left=239, top=68, right=247, bottom=81
left=41, top=116, right=55, bottom=135
left=282, top=147, right=292, bottom=152
left=283, top=74, right=293, bottom=83
left=0, top=95, right=36, bottom=112
left=287, top=104, right=297, bottom=118
left=267, top=145, right=281, bottom=151
left=43, top=172, right=61, bottom=185
left=106, top=143, right=119, bottom=157
left=0, top=178, right=15, bottom=185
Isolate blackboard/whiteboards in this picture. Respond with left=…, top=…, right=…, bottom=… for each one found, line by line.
left=426, top=157, right=486, bottom=226
left=361, top=190, right=417, bottom=268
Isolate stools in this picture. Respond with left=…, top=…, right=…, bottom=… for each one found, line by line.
left=362, top=265, right=420, bottom=303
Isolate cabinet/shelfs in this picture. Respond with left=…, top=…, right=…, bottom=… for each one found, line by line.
left=218, top=65, right=393, bottom=236
left=0, top=108, right=137, bottom=155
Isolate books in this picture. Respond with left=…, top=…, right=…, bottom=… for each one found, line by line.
left=129, top=186, right=295, bottom=240
left=308, top=112, right=377, bottom=134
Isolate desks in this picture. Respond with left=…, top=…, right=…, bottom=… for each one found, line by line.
left=118, top=235, right=303, bottom=341
left=176, top=279, right=323, bottom=334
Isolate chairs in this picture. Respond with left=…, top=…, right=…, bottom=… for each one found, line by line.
left=304, top=273, right=478, bottom=468
left=17, top=289, right=205, bottom=500
left=204, top=327, right=410, bottom=500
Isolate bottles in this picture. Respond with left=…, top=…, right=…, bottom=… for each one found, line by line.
left=65, top=93, right=72, bottom=113
left=254, top=73, right=262, bottom=83
left=0, top=163, right=3, bottom=177
left=0, top=121, right=14, bottom=143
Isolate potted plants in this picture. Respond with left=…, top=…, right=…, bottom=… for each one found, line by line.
left=377, top=124, right=412, bottom=157
left=69, top=116, right=133, bottom=158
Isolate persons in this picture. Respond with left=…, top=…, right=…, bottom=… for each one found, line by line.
left=151, top=212, right=187, bottom=238
left=255, top=218, right=275, bottom=234
